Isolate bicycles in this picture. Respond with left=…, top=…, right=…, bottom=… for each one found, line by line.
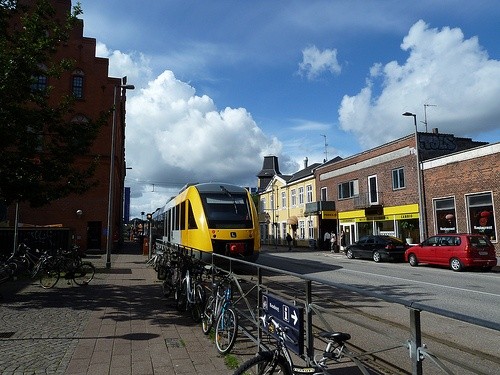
left=0, top=239, right=96, bottom=288
left=145, top=243, right=246, bottom=355
left=232, top=306, right=351, bottom=375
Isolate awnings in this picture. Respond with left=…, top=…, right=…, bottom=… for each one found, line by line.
left=287, top=216, right=297, bottom=225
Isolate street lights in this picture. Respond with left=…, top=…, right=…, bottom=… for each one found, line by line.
left=402, top=112, right=424, bottom=243
left=147, top=214, right=152, bottom=260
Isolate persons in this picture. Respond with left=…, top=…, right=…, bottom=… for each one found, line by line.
left=324, top=231, right=345, bottom=253
left=286, top=233, right=293, bottom=250
left=128, top=230, right=134, bottom=242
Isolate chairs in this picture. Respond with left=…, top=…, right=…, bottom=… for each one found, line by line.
left=438, top=240, right=450, bottom=246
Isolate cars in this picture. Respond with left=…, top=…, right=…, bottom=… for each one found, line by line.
left=344, top=235, right=408, bottom=263
left=404, top=233, right=497, bottom=272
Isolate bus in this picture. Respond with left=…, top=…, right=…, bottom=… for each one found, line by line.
left=148, top=183, right=262, bottom=273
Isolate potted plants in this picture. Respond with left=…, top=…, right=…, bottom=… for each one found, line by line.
left=401, top=222, right=416, bottom=243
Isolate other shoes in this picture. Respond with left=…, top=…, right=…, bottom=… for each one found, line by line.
left=289, top=249, right=292, bottom=250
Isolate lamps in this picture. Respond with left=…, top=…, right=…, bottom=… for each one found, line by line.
left=76, top=210, right=82, bottom=217
